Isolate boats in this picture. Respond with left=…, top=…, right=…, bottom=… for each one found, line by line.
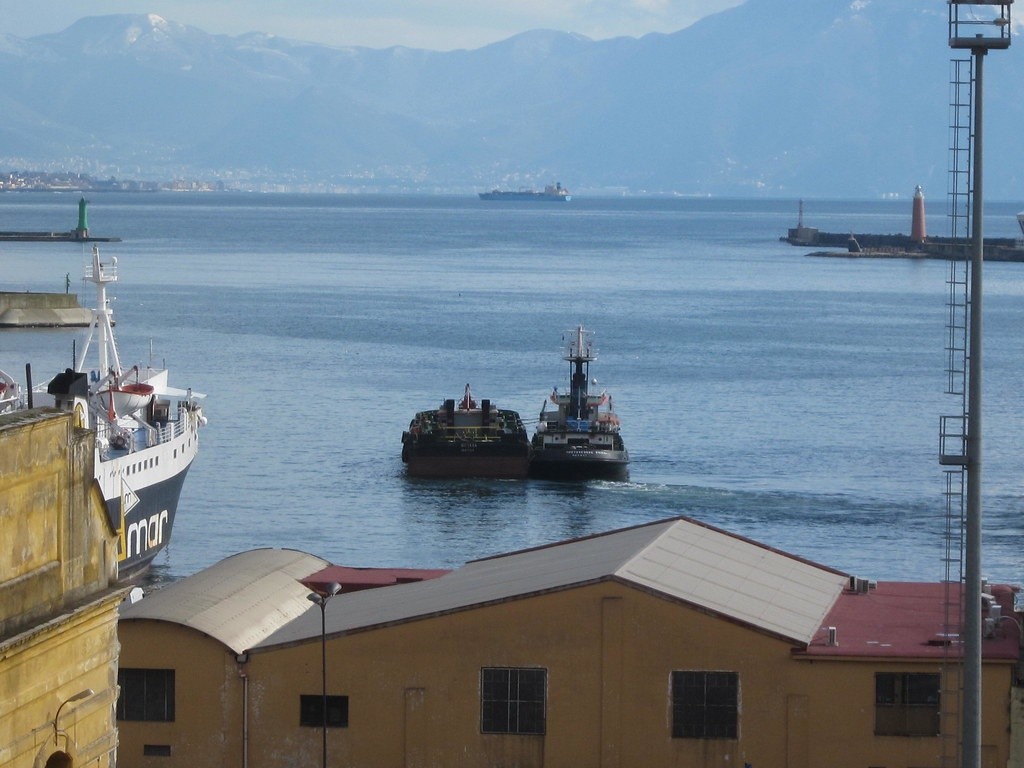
left=478, top=181, right=574, bottom=203
left=401, top=381, right=533, bottom=479
left=0, top=238, right=215, bottom=580
left=97, top=381, right=155, bottom=419
left=530, top=323, right=631, bottom=478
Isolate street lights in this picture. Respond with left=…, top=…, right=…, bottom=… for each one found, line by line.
left=306, top=579, right=345, bottom=768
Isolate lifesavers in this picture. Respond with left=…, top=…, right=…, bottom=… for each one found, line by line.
left=115, top=436, right=125, bottom=446
